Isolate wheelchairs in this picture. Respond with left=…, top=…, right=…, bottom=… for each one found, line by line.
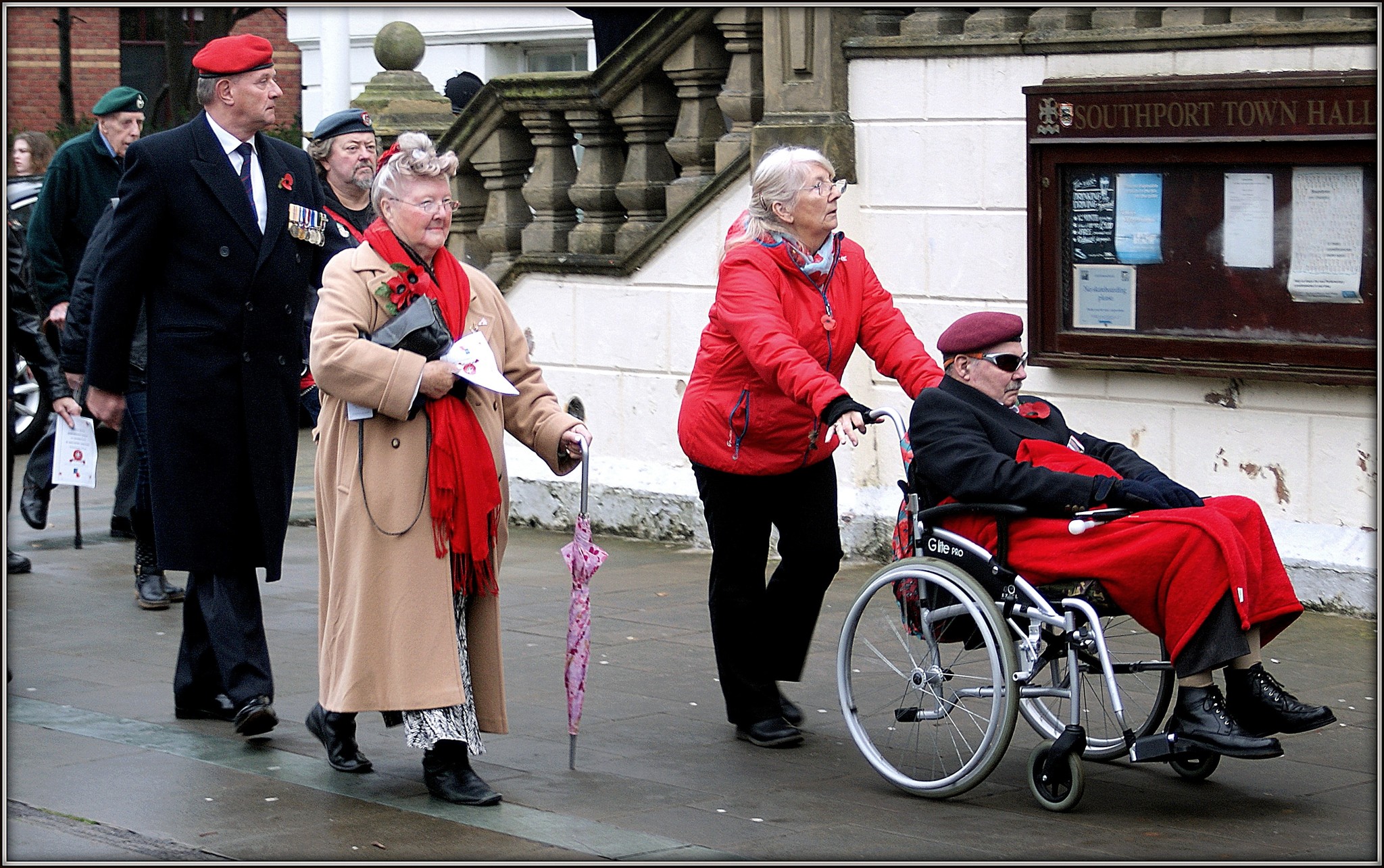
left=831, top=406, right=1222, bottom=812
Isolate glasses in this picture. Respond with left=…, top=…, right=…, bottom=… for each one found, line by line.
left=386, top=196, right=461, bottom=214
left=966, top=352, right=1029, bottom=372
left=780, top=179, right=847, bottom=196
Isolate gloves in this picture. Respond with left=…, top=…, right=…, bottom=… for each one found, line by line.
left=1135, top=470, right=1206, bottom=509
left=1093, top=474, right=1170, bottom=514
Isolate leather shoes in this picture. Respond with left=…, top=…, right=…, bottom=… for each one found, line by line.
left=304, top=703, right=371, bottom=772
left=134, top=572, right=171, bottom=608
left=778, top=690, right=804, bottom=727
left=7, top=549, right=31, bottom=574
left=111, top=513, right=139, bottom=536
left=162, top=576, right=188, bottom=601
left=232, top=696, right=279, bottom=736
left=733, top=718, right=806, bottom=748
left=172, top=696, right=236, bottom=724
left=422, top=755, right=502, bottom=805
left=20, top=477, right=51, bottom=530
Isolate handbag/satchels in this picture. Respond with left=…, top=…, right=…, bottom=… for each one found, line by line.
left=364, top=293, right=454, bottom=363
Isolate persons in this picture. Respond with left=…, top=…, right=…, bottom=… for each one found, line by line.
left=301, top=131, right=594, bottom=813
left=301, top=108, right=379, bottom=423
left=907, top=312, right=1338, bottom=760
left=442, top=69, right=484, bottom=114
left=17, top=84, right=150, bottom=541
left=56, top=33, right=349, bottom=735
left=678, top=142, right=943, bottom=754
left=6, top=130, right=83, bottom=576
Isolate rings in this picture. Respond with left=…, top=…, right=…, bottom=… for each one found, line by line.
left=834, top=423, right=840, bottom=426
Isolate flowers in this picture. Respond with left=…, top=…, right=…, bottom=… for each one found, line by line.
left=278, top=173, right=293, bottom=191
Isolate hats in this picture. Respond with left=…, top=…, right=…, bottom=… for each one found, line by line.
left=93, top=86, right=149, bottom=114
left=937, top=312, right=1023, bottom=355
left=192, top=34, right=275, bottom=78
left=312, top=109, right=376, bottom=141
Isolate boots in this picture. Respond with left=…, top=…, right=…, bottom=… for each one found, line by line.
left=1161, top=686, right=1285, bottom=759
left=1222, top=663, right=1338, bottom=738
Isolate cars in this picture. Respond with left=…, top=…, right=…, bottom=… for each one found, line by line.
left=7, top=173, right=102, bottom=455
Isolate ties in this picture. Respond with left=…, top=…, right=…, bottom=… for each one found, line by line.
left=233, top=143, right=260, bottom=222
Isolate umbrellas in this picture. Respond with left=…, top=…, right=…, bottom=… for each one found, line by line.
left=559, top=433, right=609, bottom=773
